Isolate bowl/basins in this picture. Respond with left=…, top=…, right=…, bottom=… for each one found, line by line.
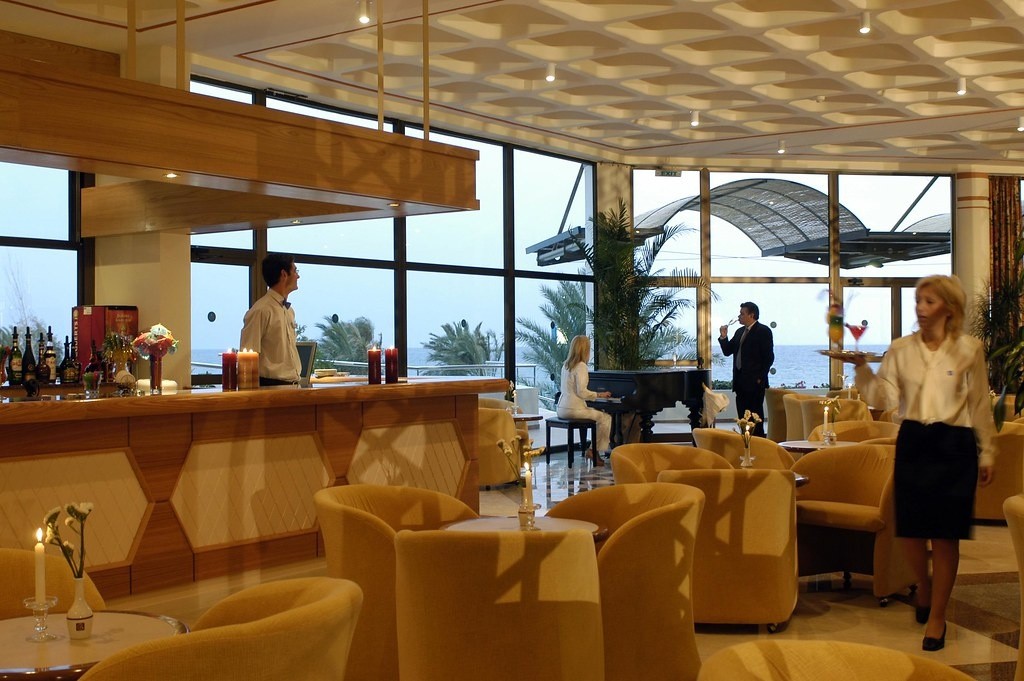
left=314, top=368, right=338, bottom=377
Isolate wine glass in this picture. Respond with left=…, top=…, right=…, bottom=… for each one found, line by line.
left=724, top=315, right=739, bottom=328
left=847, top=325, right=867, bottom=354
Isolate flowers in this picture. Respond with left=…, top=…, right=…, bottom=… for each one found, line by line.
left=495, top=434, right=545, bottom=487
left=504, top=380, right=516, bottom=403
left=103, top=328, right=135, bottom=363
left=732, top=410, right=762, bottom=448
left=44, top=502, right=94, bottom=578
left=818, top=394, right=843, bottom=423
left=132, top=324, right=181, bottom=359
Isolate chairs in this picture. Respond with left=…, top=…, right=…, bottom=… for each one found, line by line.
left=0, top=397, right=1024, bottom=681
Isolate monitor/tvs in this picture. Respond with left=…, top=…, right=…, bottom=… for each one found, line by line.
left=295, top=341, right=317, bottom=387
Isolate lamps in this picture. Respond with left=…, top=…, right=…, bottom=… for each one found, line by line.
left=956, top=76, right=967, bottom=96
left=545, top=63, right=555, bottom=82
left=860, top=11, right=870, bottom=33
left=690, top=111, right=699, bottom=126
left=778, top=139, right=785, bottom=153
left=1017, top=116, right=1024, bottom=132
left=359, top=0, right=371, bottom=24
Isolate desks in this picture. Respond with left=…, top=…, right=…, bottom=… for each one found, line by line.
left=0, top=609, right=190, bottom=681
left=513, top=414, right=543, bottom=424
left=437, top=515, right=609, bottom=542
left=778, top=440, right=858, bottom=455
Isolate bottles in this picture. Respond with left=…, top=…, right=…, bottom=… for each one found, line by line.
left=7, top=325, right=101, bottom=384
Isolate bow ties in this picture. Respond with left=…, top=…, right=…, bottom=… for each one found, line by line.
left=282, top=298, right=291, bottom=310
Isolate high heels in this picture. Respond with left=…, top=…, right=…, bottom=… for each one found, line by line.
left=585, top=448, right=604, bottom=467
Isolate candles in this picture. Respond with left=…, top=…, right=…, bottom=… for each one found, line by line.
left=526, top=468, right=531, bottom=508
left=745, top=431, right=750, bottom=459
left=385, top=348, right=398, bottom=383
left=514, top=394, right=517, bottom=405
left=222, top=352, right=259, bottom=390
left=823, top=410, right=828, bottom=431
left=35, top=540, right=46, bottom=604
left=368, top=349, right=382, bottom=384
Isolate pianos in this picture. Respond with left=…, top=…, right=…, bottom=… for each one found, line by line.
left=555, top=368, right=712, bottom=450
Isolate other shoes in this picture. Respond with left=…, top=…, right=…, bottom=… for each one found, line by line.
left=914, top=598, right=930, bottom=624
left=922, top=622, right=947, bottom=652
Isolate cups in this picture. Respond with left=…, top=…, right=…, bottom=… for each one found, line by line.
left=83, top=377, right=101, bottom=399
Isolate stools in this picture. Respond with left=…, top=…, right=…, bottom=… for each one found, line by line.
left=545, top=417, right=597, bottom=468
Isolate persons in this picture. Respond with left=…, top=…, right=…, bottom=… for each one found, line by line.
left=718, top=301, right=774, bottom=439
left=557, top=335, right=612, bottom=467
left=239, top=253, right=302, bottom=387
left=828, top=276, right=998, bottom=652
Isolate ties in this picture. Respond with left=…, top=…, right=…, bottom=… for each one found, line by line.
left=736, top=328, right=749, bottom=370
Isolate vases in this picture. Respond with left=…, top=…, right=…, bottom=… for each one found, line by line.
left=149, top=354, right=162, bottom=395
left=518, top=488, right=534, bottom=527
left=114, top=353, right=130, bottom=375
left=825, top=422, right=837, bottom=446
left=66, top=577, right=93, bottom=639
left=740, top=448, right=752, bottom=469
left=506, top=402, right=512, bottom=414
